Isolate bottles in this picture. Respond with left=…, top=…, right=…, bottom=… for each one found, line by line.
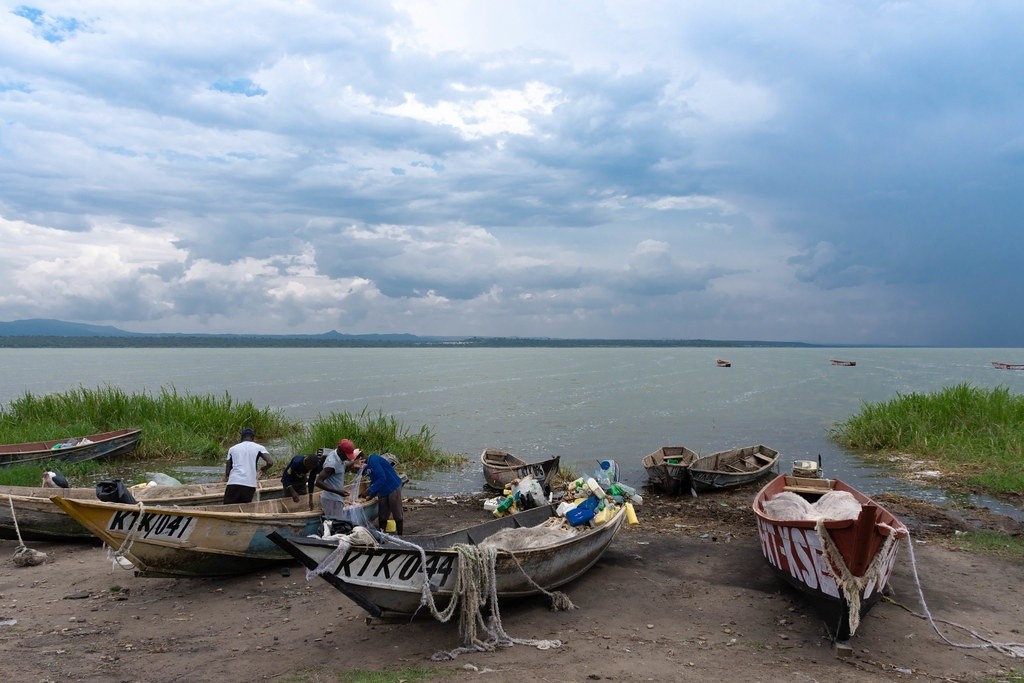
left=549, top=459, right=643, bottom=527
left=691, top=488, right=697, bottom=497
left=492, top=478, right=538, bottom=518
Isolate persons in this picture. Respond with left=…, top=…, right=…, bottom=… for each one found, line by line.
left=281, top=438, right=403, bottom=536
left=223, top=428, right=274, bottom=504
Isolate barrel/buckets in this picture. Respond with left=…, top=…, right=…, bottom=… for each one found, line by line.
left=385, top=520, right=396, bottom=532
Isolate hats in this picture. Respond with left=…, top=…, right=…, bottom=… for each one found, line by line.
left=309, top=455, right=318, bottom=465
left=338, top=439, right=355, bottom=460
left=241, top=428, right=255, bottom=436
left=346, top=449, right=363, bottom=469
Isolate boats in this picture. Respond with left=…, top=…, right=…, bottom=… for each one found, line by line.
left=49, top=472, right=409, bottom=580
left=716, top=359, right=731, bottom=367
left=991, top=362, right=1024, bottom=371
left=687, top=443, right=781, bottom=490
left=829, top=359, right=857, bottom=367
left=753, top=459, right=908, bottom=641
left=641, top=446, right=698, bottom=490
left=0, top=427, right=144, bottom=466
left=481, top=447, right=561, bottom=497
left=0, top=453, right=400, bottom=545
left=264, top=459, right=632, bottom=624
